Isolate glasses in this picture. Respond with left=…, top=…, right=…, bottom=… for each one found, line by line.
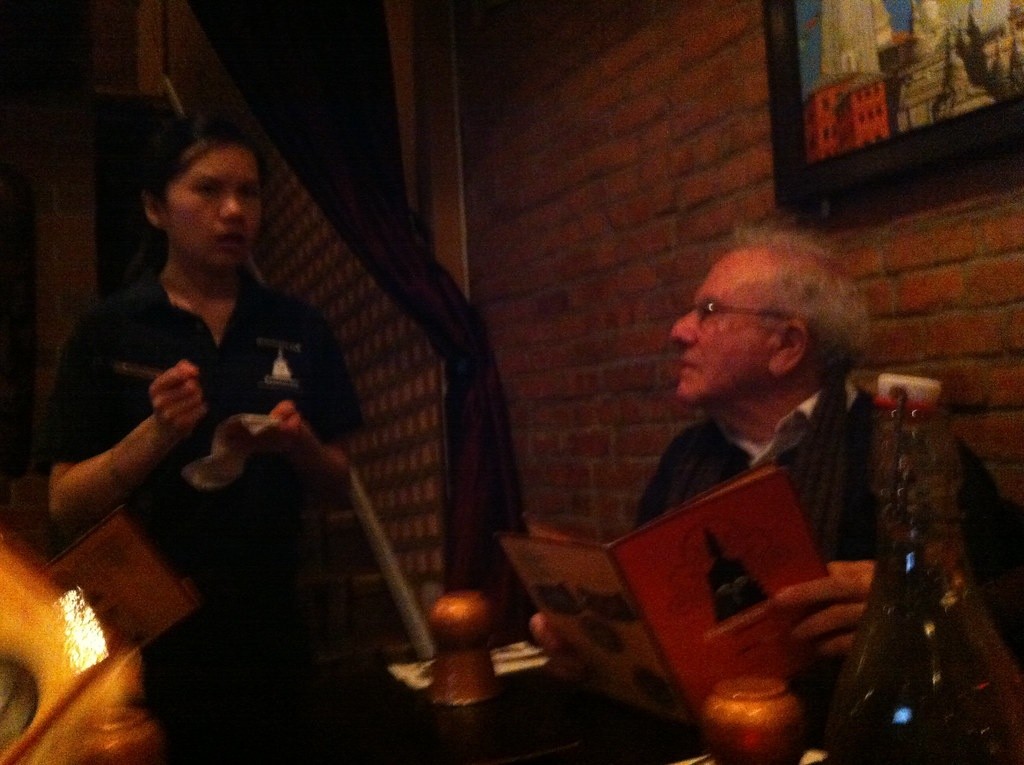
left=696, top=299, right=782, bottom=325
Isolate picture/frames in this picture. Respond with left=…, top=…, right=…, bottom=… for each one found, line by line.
left=761, top=0, right=1024, bottom=221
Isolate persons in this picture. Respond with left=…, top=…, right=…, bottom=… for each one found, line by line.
left=531, top=227, right=1024, bottom=765
left=40, top=114, right=365, bottom=764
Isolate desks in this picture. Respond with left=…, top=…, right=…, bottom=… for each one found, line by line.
left=372, top=641, right=640, bottom=765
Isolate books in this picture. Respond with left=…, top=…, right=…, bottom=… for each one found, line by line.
left=500, top=459, right=842, bottom=728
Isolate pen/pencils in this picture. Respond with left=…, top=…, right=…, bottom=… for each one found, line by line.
left=114, top=362, right=161, bottom=375
